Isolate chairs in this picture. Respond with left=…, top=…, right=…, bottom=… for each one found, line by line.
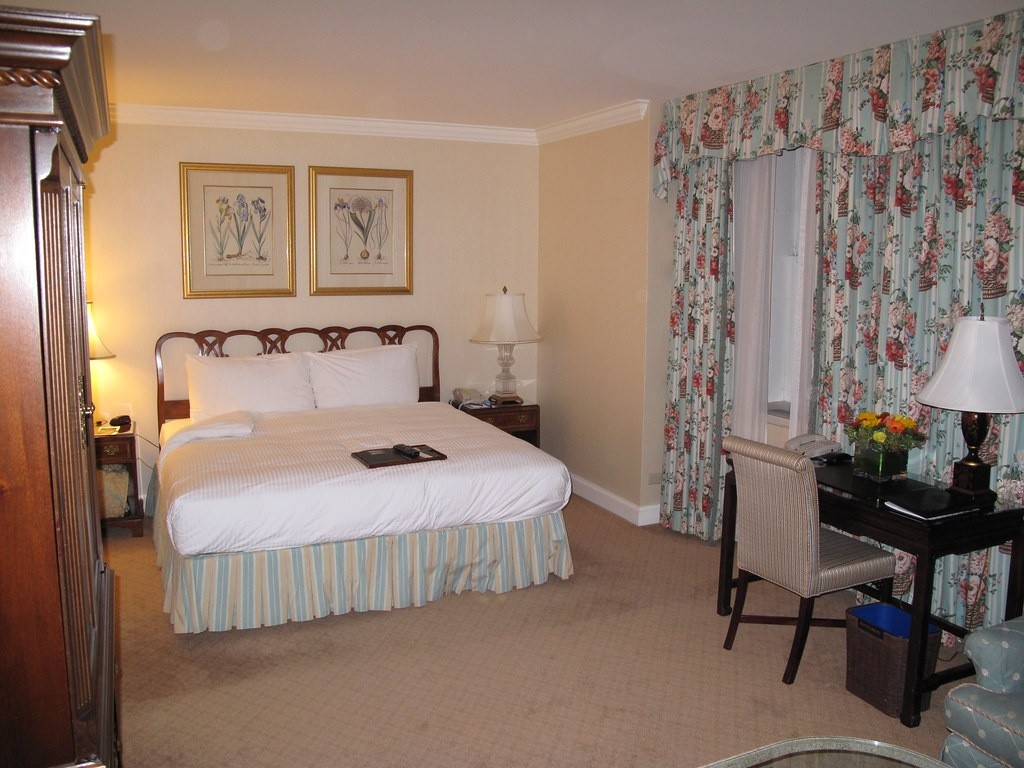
left=717, top=435, right=897, bottom=685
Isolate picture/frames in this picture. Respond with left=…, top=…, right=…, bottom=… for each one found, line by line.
left=309, top=165, right=413, bottom=296
left=180, top=162, right=296, bottom=299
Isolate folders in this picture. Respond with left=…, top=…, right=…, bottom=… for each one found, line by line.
left=879, top=487, right=983, bottom=524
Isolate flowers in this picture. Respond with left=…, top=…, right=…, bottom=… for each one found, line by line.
left=836, top=411, right=930, bottom=452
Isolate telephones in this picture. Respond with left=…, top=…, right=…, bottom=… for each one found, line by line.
left=783, top=433, right=839, bottom=457
left=452, top=387, right=488, bottom=404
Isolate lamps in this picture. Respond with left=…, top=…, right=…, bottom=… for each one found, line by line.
left=469, top=286, right=544, bottom=405
left=86, top=302, right=116, bottom=360
left=914, top=303, right=1024, bottom=504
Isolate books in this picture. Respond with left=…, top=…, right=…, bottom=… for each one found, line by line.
left=884, top=487, right=984, bottom=522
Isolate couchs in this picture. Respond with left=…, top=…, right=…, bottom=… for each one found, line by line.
left=940, top=604, right=1024, bottom=768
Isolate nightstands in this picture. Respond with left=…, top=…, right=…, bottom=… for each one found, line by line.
left=449, top=400, right=540, bottom=448
left=93, top=421, right=144, bottom=537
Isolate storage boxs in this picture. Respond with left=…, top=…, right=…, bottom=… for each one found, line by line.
left=845, top=602, right=943, bottom=717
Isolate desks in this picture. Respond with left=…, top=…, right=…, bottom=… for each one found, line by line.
left=717, top=455, right=1024, bottom=728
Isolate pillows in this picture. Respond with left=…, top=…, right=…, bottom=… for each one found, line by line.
left=185, top=353, right=316, bottom=423
left=303, top=340, right=420, bottom=409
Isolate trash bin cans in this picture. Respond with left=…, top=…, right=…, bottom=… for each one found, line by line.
left=845, top=603, right=942, bottom=716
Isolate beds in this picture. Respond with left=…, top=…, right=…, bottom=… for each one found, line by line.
left=155, top=325, right=575, bottom=634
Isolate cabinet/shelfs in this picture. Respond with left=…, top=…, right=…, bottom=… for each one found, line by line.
left=0, top=5, right=118, bottom=768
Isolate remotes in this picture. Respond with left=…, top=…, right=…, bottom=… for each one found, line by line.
left=394, top=443, right=420, bottom=458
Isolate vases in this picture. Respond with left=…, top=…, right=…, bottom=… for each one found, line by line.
left=855, top=440, right=908, bottom=482
